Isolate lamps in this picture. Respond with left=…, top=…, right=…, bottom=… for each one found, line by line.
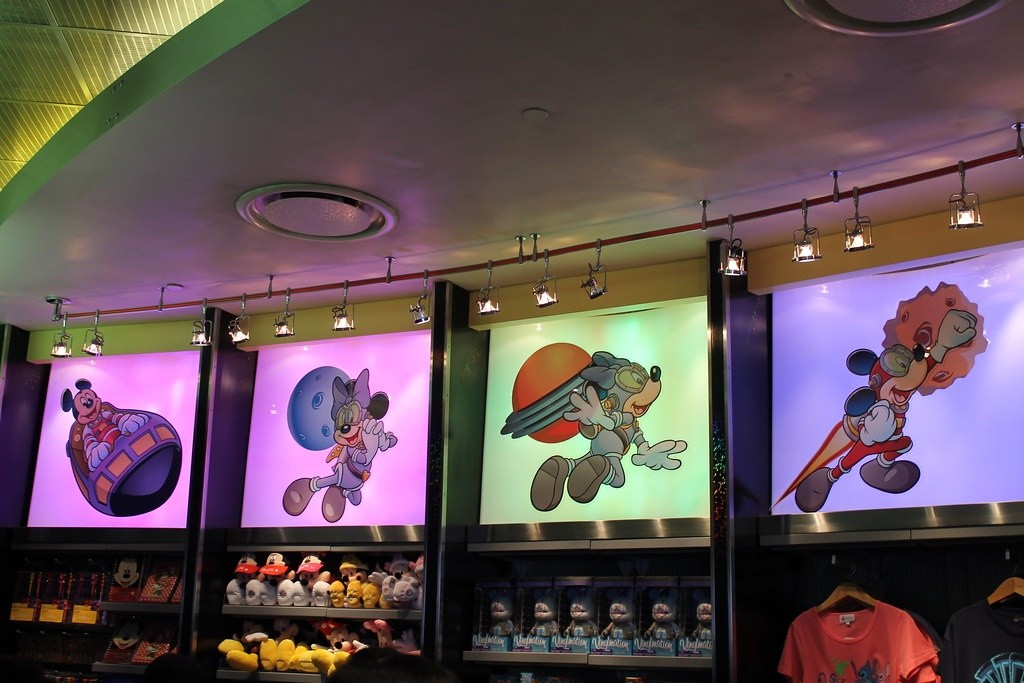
left=189, top=298, right=213, bottom=346
left=332, top=279, right=356, bottom=331
left=408, top=269, right=431, bottom=326
left=718, top=214, right=745, bottom=277
left=532, top=248, right=559, bottom=308
left=476, top=259, right=501, bottom=317
left=843, top=186, right=875, bottom=253
left=791, top=198, right=823, bottom=263
left=49, top=311, right=73, bottom=358
left=81, top=308, right=104, bottom=357
left=579, top=238, right=609, bottom=301
left=227, top=292, right=251, bottom=345
left=948, top=160, right=984, bottom=230
left=272, top=286, right=296, bottom=338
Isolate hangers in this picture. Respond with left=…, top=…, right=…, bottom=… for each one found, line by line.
left=987, top=550, right=1024, bottom=604
left=816, top=555, right=876, bottom=613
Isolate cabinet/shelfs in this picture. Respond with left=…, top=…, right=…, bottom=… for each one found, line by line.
left=215, top=543, right=425, bottom=683
left=462, top=536, right=731, bottom=683
left=91, top=541, right=187, bottom=675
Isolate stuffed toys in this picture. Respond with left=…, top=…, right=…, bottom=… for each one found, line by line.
left=294, top=554, right=334, bottom=606
left=260, top=617, right=306, bottom=672
left=311, top=618, right=376, bottom=676
left=218, top=618, right=281, bottom=670
left=362, top=619, right=421, bottom=655
left=289, top=617, right=330, bottom=673
left=368, top=552, right=424, bottom=609
left=330, top=554, right=369, bottom=608
left=227, top=553, right=295, bottom=605
left=489, top=594, right=712, bottom=643
